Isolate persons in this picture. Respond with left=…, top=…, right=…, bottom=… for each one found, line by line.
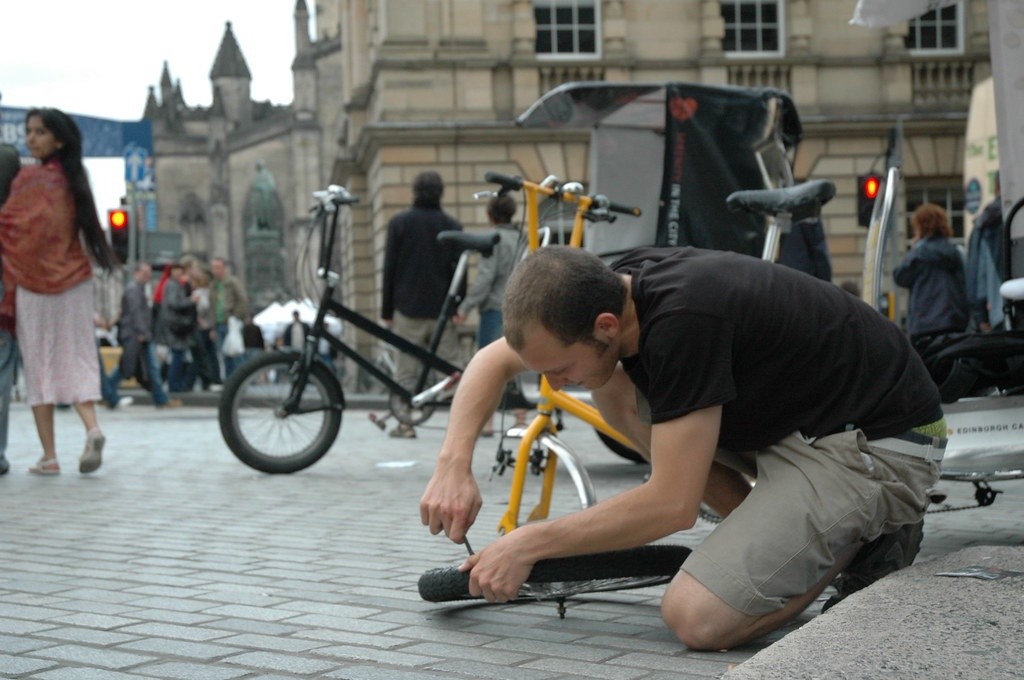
left=281, top=311, right=310, bottom=387
left=0, top=94, right=117, bottom=475
left=316, top=324, right=339, bottom=377
left=241, top=313, right=264, bottom=386
left=420, top=244, right=949, bottom=651
left=380, top=172, right=468, bottom=437
left=892, top=204, right=968, bottom=344
left=57, top=253, right=243, bottom=411
left=451, top=196, right=529, bottom=437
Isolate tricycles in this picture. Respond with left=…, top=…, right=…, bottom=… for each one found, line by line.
left=215, top=80, right=834, bottom=475
left=473, top=165, right=1023, bottom=535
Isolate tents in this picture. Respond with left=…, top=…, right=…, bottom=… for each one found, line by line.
left=252, top=297, right=343, bottom=387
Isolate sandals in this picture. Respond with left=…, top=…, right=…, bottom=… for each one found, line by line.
left=80, top=427, right=105, bottom=474
left=821, top=519, right=926, bottom=614
left=28, top=457, right=61, bottom=475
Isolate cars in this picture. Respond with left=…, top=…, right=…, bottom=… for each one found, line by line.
left=88, top=329, right=172, bottom=389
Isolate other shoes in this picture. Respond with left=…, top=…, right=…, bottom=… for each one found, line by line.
left=159, top=398, right=182, bottom=410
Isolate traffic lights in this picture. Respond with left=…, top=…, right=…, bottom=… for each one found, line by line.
left=108, top=209, right=130, bottom=263
left=854, top=173, right=883, bottom=227
